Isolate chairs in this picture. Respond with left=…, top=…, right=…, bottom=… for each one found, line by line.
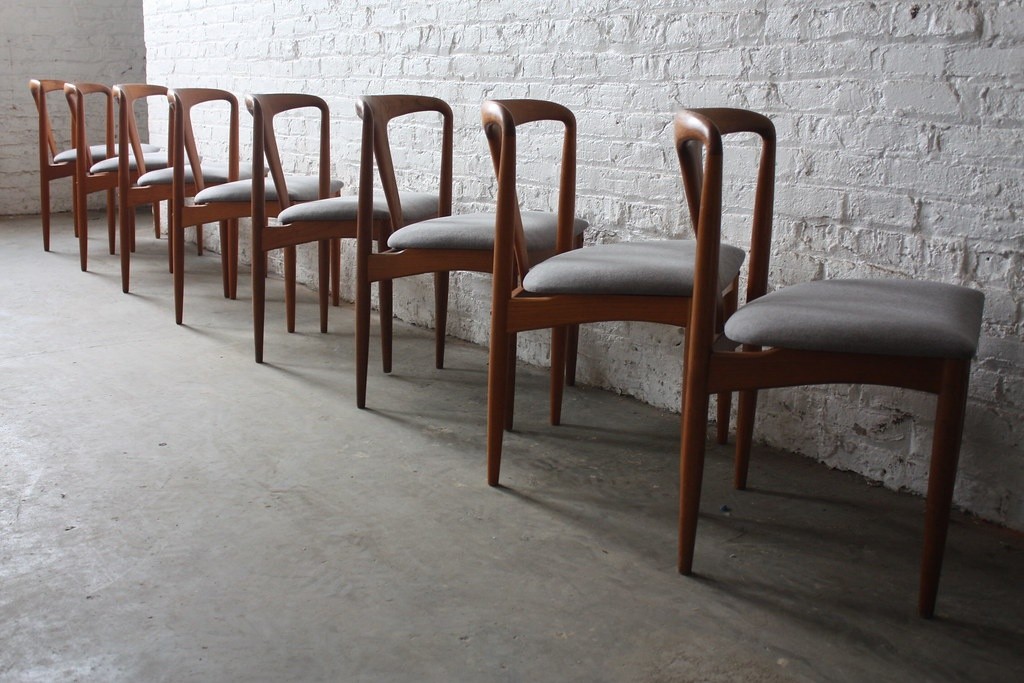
left=29, top=78, right=161, bottom=255
left=64, top=82, right=203, bottom=274
left=168, top=87, right=344, bottom=334
left=113, top=82, right=269, bottom=300
left=482, top=100, right=744, bottom=488
left=675, top=107, right=985, bottom=617
left=245, top=93, right=439, bottom=375
left=355, top=95, right=590, bottom=432
